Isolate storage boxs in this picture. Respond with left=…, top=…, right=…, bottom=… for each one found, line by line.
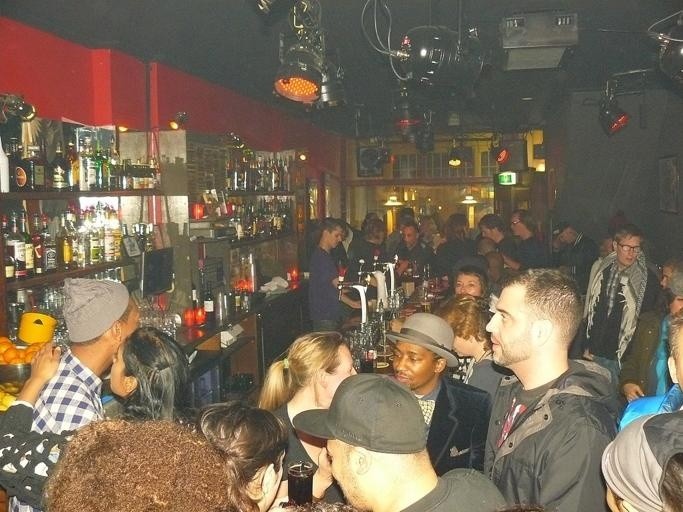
left=221, top=324, right=246, bottom=350
left=189, top=366, right=223, bottom=425
left=196, top=335, right=223, bottom=353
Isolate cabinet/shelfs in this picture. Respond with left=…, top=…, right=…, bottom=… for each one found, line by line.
left=187, top=137, right=304, bottom=424
left=1, top=107, right=190, bottom=428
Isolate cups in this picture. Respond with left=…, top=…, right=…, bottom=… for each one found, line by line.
left=343, top=304, right=389, bottom=375
left=393, top=259, right=436, bottom=315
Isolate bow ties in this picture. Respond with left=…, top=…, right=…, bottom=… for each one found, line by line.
left=417, top=399, right=435, bottom=424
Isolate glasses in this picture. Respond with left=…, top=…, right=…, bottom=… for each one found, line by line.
left=510, top=221, right=520, bottom=225
left=617, top=243, right=642, bottom=253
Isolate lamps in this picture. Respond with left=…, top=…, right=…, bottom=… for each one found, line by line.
left=376, top=135, right=512, bottom=167
left=2, top=92, right=37, bottom=127
left=596, top=67, right=666, bottom=138
left=258, top=1, right=350, bottom=113
left=390, top=76, right=435, bottom=155
left=223, top=129, right=246, bottom=154
left=296, top=146, right=313, bottom=168
left=171, top=109, right=187, bottom=132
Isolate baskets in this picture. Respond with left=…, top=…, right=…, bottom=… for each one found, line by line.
left=199, top=258, right=224, bottom=287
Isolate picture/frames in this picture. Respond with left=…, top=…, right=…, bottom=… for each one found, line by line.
left=656, top=153, right=680, bottom=216
left=356, top=144, right=384, bottom=177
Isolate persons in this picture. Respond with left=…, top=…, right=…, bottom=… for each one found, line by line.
left=1, top=207, right=682, bottom=512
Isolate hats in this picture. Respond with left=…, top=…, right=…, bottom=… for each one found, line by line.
left=600, top=414, right=683, bottom=512
left=386, top=312, right=459, bottom=367
left=552, top=222, right=570, bottom=239
left=63, top=278, right=130, bottom=343
left=670, top=272, right=683, bottom=296
left=293, top=372, right=426, bottom=453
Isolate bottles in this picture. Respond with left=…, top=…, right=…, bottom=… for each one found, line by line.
left=5, top=212, right=140, bottom=276
left=231, top=200, right=294, bottom=237
left=229, top=157, right=290, bottom=191
left=195, top=277, right=253, bottom=322
left=6, top=140, right=159, bottom=192
